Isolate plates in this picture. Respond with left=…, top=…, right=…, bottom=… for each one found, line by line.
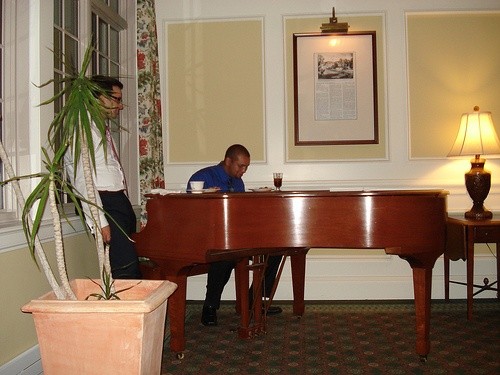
left=253, top=189, right=271, bottom=192
left=203, top=189, right=221, bottom=192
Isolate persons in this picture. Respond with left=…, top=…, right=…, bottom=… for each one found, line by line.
left=64, top=75, right=141, bottom=280
left=186, top=144, right=283, bottom=327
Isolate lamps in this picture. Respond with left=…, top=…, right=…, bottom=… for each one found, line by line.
left=446, top=106, right=500, bottom=221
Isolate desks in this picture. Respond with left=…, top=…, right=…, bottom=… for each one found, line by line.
left=445, top=212, right=500, bottom=321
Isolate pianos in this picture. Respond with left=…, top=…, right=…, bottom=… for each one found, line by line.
left=131, top=189, right=450, bottom=363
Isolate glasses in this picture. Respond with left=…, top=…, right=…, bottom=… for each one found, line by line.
left=107, top=94, right=120, bottom=101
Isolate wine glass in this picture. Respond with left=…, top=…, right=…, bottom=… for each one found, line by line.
left=273, top=172, right=282, bottom=192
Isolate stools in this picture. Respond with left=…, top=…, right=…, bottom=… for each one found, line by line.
left=141, top=260, right=241, bottom=315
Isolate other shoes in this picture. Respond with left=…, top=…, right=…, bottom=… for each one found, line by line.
left=201, top=299, right=217, bottom=325
left=249, top=300, right=283, bottom=313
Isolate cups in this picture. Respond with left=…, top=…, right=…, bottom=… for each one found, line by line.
left=190, top=180, right=204, bottom=194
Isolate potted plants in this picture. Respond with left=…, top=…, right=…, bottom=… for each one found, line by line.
left=0, top=37, right=178, bottom=375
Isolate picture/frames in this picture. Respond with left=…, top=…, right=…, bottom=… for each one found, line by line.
left=293, top=30, right=379, bottom=146
